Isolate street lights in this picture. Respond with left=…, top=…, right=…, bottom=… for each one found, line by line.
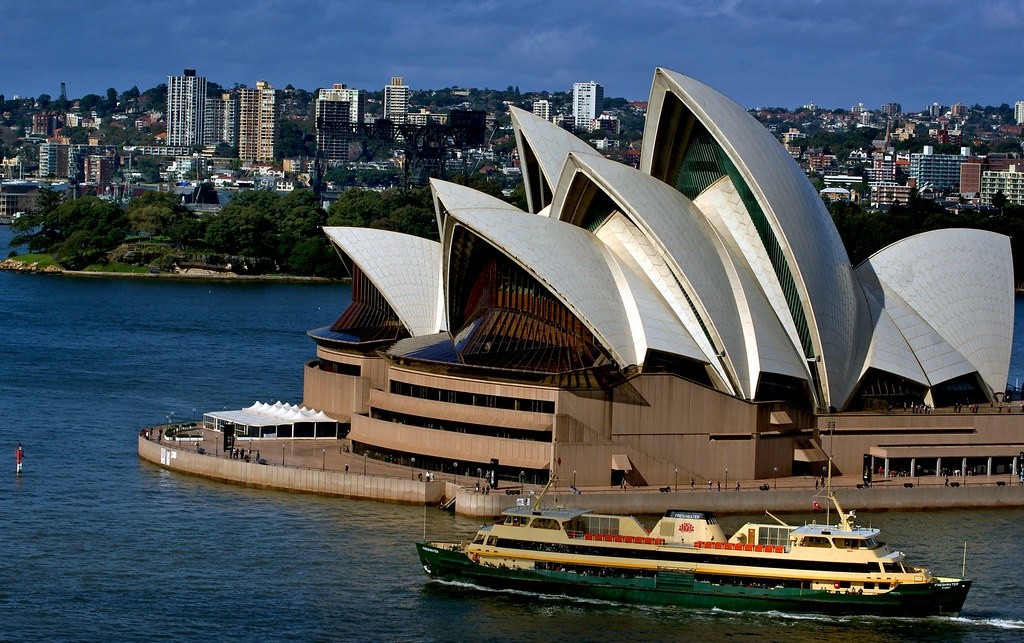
left=170, top=411, right=179, bottom=428
left=282, top=445, right=285, bottom=466
left=518, top=470, right=524, bottom=493
left=675, top=469, right=678, bottom=492
left=166, top=415, right=169, bottom=429
left=453, top=462, right=457, bottom=484
left=573, top=470, right=576, bottom=487
left=248, top=439, right=252, bottom=460
left=363, top=454, right=367, bottom=476
left=773, top=467, right=778, bottom=489
left=192, top=409, right=196, bottom=424
left=411, top=458, right=416, bottom=480
left=214, top=434, right=219, bottom=457
left=725, top=468, right=727, bottom=490
left=477, top=467, right=482, bottom=489
left=322, top=449, right=326, bottom=471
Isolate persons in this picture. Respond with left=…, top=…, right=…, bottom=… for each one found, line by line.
left=717, top=481, right=720, bottom=491
left=346, top=464, right=349, bottom=473
left=141, top=428, right=163, bottom=443
left=736, top=481, right=740, bottom=491
left=691, top=477, right=694, bottom=488
left=915, top=398, right=1024, bottom=414
left=820, top=476, right=825, bottom=485
left=476, top=480, right=480, bottom=492
left=879, top=466, right=884, bottom=476
left=944, top=474, right=949, bottom=487
left=486, top=470, right=490, bottom=482
left=708, top=480, right=712, bottom=490
left=229, top=448, right=244, bottom=459
left=762, top=483, right=769, bottom=491
left=418, top=471, right=434, bottom=481
left=196, top=442, right=199, bottom=450
left=473, top=543, right=865, bottom=596
left=481, top=485, right=490, bottom=495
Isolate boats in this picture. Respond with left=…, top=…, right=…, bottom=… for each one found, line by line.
left=417, top=457, right=972, bottom=618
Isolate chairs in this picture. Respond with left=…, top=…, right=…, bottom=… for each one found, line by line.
left=694, top=541, right=785, bottom=553
left=569, top=531, right=665, bottom=545
